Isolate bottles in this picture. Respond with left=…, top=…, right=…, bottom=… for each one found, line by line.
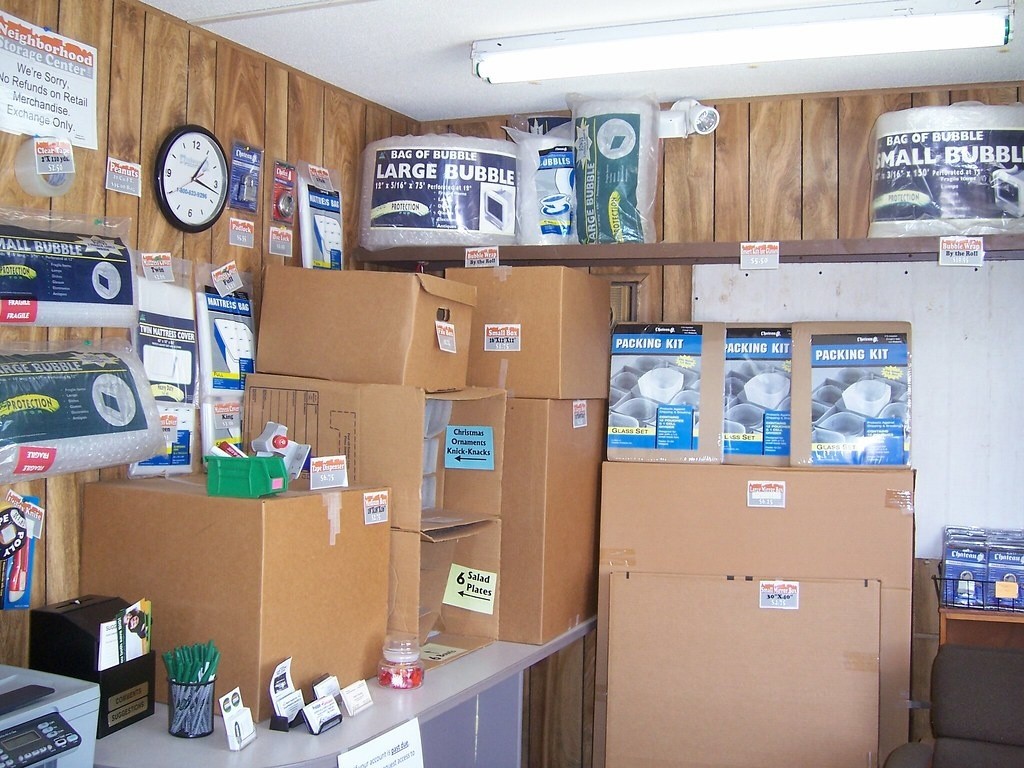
left=376, top=635, right=425, bottom=691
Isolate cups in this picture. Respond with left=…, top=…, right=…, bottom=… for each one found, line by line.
left=724, top=367, right=789, bottom=436
left=610, top=358, right=700, bottom=435
left=812, top=366, right=906, bottom=447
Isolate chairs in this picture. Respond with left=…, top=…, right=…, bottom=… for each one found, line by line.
left=881, top=641, right=1024, bottom=768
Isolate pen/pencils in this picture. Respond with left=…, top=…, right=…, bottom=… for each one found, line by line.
left=162, top=638, right=222, bottom=738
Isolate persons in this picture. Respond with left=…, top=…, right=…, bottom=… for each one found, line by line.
left=129, top=612, right=152, bottom=638
left=122, top=608, right=146, bottom=633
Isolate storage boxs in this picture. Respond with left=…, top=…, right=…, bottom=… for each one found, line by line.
left=495, top=399, right=611, bottom=647
left=242, top=371, right=508, bottom=535
left=438, top=263, right=614, bottom=401
left=388, top=517, right=502, bottom=674
left=75, top=469, right=393, bottom=729
left=590, top=459, right=915, bottom=766
left=250, top=264, right=480, bottom=396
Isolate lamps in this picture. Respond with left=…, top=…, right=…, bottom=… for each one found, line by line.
left=656, top=97, right=722, bottom=139
left=468, top=0, right=1014, bottom=86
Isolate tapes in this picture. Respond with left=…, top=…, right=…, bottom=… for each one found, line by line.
left=14, top=140, right=73, bottom=199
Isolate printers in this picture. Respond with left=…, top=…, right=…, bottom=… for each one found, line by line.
left=0, top=663, right=100, bottom=768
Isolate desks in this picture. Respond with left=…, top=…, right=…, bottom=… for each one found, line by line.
left=94, top=617, right=599, bottom=768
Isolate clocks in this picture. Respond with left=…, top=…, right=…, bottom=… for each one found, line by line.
left=153, top=123, right=231, bottom=236
